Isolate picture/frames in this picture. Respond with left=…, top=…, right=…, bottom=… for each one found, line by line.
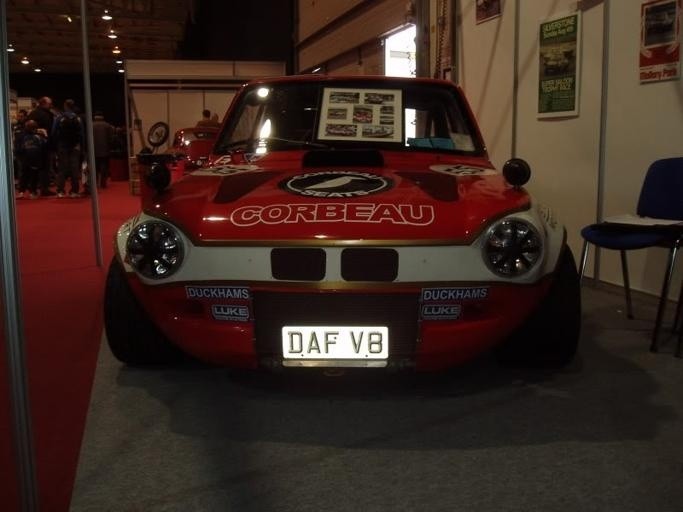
left=537, top=10, right=583, bottom=120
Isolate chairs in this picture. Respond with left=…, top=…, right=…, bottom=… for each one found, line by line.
left=576, top=157, right=683, bottom=354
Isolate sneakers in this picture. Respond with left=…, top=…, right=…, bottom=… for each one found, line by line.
left=15, top=189, right=89, bottom=199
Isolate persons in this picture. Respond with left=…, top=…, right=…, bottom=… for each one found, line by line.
left=12, top=96, right=123, bottom=199
left=196, top=110, right=219, bottom=127
